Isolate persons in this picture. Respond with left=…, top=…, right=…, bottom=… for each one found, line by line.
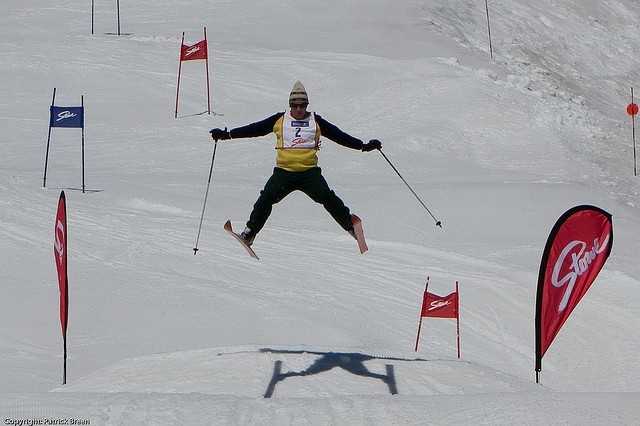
left=207, top=80, right=382, bottom=248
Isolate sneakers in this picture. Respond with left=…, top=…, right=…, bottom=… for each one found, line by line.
left=238, top=227, right=255, bottom=246
left=348, top=227, right=357, bottom=240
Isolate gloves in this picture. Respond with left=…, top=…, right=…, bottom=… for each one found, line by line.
left=362, top=139, right=382, bottom=152
left=209, top=127, right=230, bottom=141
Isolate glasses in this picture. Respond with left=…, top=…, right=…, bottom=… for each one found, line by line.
left=289, top=102, right=308, bottom=108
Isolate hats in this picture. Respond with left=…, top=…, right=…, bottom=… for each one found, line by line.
left=289, top=80, right=308, bottom=103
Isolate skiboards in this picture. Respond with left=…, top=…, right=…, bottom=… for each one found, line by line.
left=224, top=214, right=368, bottom=260
